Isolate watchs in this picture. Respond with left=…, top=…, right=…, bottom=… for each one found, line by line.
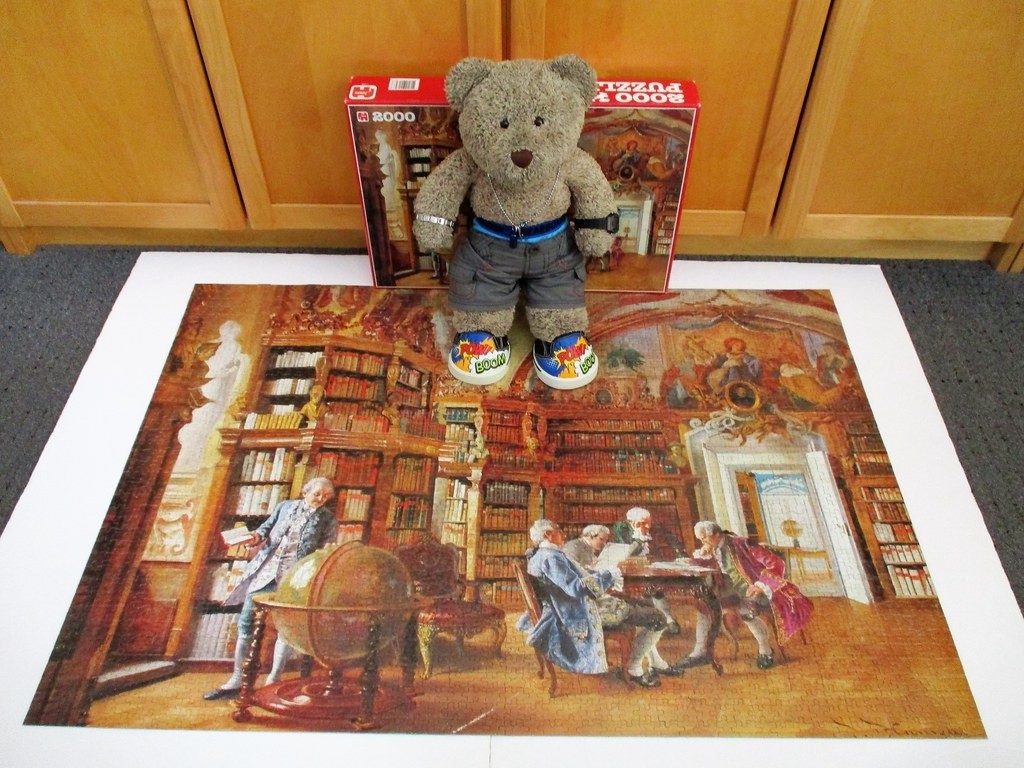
left=574, top=213, right=620, bottom=235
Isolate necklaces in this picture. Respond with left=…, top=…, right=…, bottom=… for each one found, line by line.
left=486, top=163, right=562, bottom=237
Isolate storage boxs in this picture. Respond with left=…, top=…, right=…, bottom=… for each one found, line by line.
left=343, top=75, right=701, bottom=295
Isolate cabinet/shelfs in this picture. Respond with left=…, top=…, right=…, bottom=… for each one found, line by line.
left=0, top=0, right=508, bottom=257
left=507, top=0, right=1024, bottom=273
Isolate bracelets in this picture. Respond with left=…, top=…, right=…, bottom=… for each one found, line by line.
left=417, top=213, right=456, bottom=228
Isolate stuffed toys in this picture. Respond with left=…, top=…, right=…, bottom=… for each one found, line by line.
left=410, top=54, right=621, bottom=391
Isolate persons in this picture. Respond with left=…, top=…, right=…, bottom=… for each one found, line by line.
left=560, top=522, right=611, bottom=568
left=514, top=518, right=685, bottom=690
left=609, top=505, right=687, bottom=637
left=671, top=520, right=817, bottom=671
left=202, top=476, right=340, bottom=701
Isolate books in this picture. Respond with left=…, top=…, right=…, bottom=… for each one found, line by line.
left=549, top=480, right=685, bottom=546
left=189, top=445, right=301, bottom=662
left=843, top=418, right=938, bottom=600
left=481, top=407, right=682, bottom=475
left=304, top=449, right=473, bottom=576
left=476, top=479, right=529, bottom=609
left=241, top=345, right=477, bottom=462
